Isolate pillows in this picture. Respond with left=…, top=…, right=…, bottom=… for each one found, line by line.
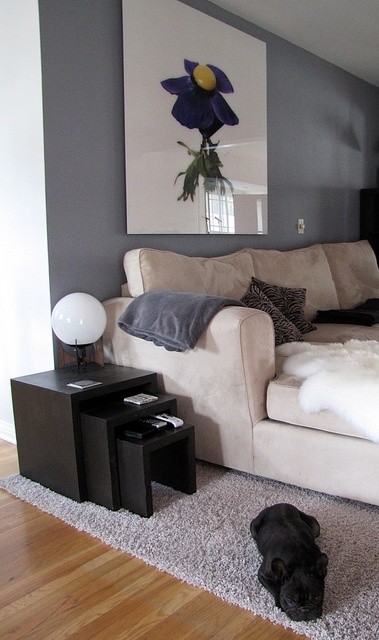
left=251, top=277, right=317, bottom=336
left=243, top=243, right=341, bottom=324
left=319, top=238, right=379, bottom=309
left=123, top=247, right=255, bottom=310
left=240, top=282, right=304, bottom=346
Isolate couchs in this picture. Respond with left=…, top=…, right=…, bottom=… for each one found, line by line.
left=93, top=239, right=379, bottom=507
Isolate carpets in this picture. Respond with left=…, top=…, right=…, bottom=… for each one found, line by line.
left=1, top=461, right=379, bottom=640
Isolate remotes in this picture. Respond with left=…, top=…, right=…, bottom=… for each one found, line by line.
left=139, top=418, right=168, bottom=429
left=154, top=413, right=184, bottom=428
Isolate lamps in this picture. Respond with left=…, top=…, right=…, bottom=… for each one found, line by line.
left=51, top=292, right=108, bottom=374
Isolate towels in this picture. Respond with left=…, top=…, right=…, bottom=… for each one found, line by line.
left=118, top=290, right=248, bottom=352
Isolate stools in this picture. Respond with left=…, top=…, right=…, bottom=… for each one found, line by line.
left=80, top=389, right=177, bottom=510
left=117, top=423, right=197, bottom=518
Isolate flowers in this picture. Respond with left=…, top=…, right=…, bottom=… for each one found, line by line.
left=161, top=59, right=239, bottom=202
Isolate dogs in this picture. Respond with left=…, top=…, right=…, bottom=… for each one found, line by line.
left=250, top=503, right=328, bottom=622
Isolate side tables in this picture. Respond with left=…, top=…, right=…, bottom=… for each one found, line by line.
left=10, top=361, right=158, bottom=504
